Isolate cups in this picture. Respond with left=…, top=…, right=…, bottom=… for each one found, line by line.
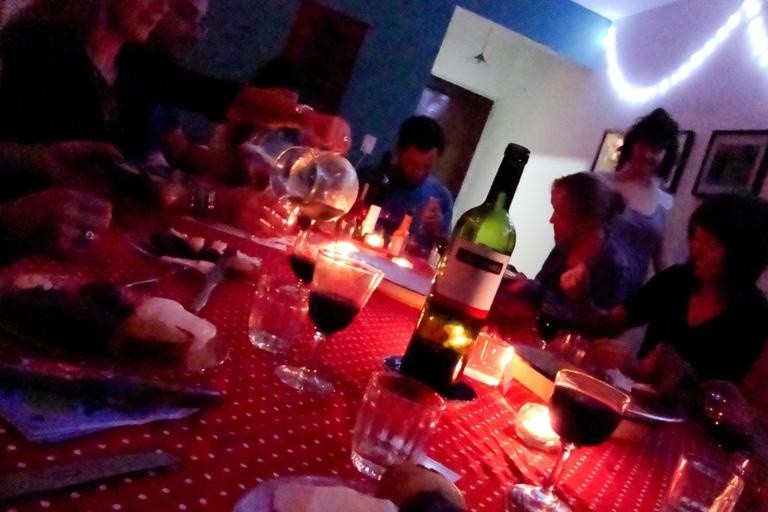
left=558, top=330, right=590, bottom=368
left=248, top=260, right=313, bottom=361
left=349, top=367, right=445, bottom=480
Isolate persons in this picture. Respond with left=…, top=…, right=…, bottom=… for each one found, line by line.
left=355, top=115, right=453, bottom=237
left=373, top=463, right=470, bottom=512
left=560, top=182, right=768, bottom=391
left=600, top=107, right=681, bottom=308
left=0, top=0, right=354, bottom=257
left=504, top=170, right=639, bottom=323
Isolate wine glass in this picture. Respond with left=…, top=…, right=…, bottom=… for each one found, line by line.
left=699, top=380, right=731, bottom=428
left=291, top=219, right=357, bottom=293
left=534, top=290, right=561, bottom=349
left=507, top=368, right=630, bottom=511
left=274, top=246, right=385, bottom=393
left=277, top=150, right=360, bottom=262
left=667, top=451, right=737, bottom=510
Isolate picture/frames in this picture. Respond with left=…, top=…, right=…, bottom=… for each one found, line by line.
left=591, top=126, right=768, bottom=201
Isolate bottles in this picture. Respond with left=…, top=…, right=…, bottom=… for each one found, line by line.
left=388, top=212, right=413, bottom=257
left=419, top=195, right=438, bottom=238
left=396, top=136, right=530, bottom=391
